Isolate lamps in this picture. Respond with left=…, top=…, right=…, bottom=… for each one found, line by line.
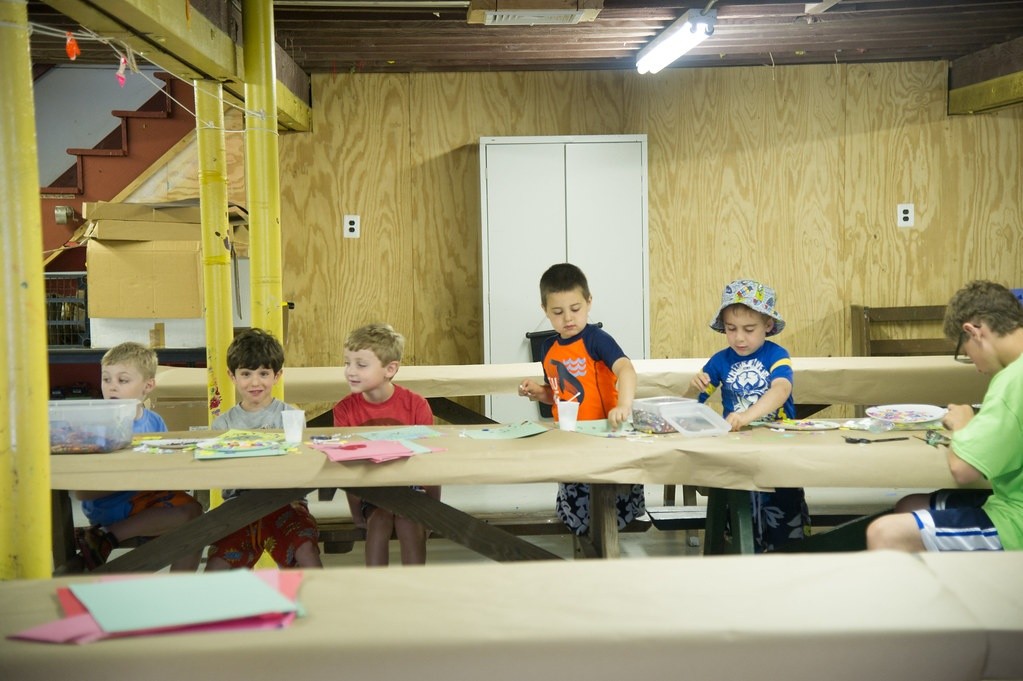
left=636, top=9, right=717, bottom=74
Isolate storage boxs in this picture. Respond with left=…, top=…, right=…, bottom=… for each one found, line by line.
left=632, top=395, right=732, bottom=438
left=81, top=202, right=205, bottom=240
left=49, top=400, right=140, bottom=454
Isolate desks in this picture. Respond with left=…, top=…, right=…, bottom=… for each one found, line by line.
left=0, top=342, right=1023, bottom=681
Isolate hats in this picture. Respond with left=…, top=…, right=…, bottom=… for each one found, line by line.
left=710, top=280, right=785, bottom=336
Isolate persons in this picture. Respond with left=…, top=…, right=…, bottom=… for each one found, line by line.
left=204, top=328, right=324, bottom=571
left=518, top=264, right=638, bottom=559
left=74, top=342, right=204, bottom=573
left=867, top=279, right=1023, bottom=553
left=691, top=279, right=812, bottom=555
left=332, top=323, right=434, bottom=566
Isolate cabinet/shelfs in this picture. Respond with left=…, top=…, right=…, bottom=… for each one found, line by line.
left=479, top=134, right=650, bottom=422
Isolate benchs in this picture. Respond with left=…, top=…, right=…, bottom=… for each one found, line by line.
left=648, top=509, right=882, bottom=528
left=851, top=305, right=974, bottom=417
left=72, top=510, right=652, bottom=555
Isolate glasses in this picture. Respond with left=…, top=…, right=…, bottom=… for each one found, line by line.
left=954, top=325, right=982, bottom=364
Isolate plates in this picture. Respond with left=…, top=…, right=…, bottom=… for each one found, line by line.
left=197, top=439, right=274, bottom=452
left=143, top=439, right=206, bottom=448
left=866, top=404, right=945, bottom=423
left=769, top=421, right=839, bottom=431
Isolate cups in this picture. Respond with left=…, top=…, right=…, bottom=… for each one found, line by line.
left=279, top=410, right=305, bottom=442
left=558, top=402, right=580, bottom=431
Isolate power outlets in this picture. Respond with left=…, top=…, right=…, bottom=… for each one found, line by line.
left=343, top=215, right=360, bottom=238
left=898, top=204, right=915, bottom=228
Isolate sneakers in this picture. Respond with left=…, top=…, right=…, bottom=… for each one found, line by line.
left=74, top=523, right=120, bottom=570
